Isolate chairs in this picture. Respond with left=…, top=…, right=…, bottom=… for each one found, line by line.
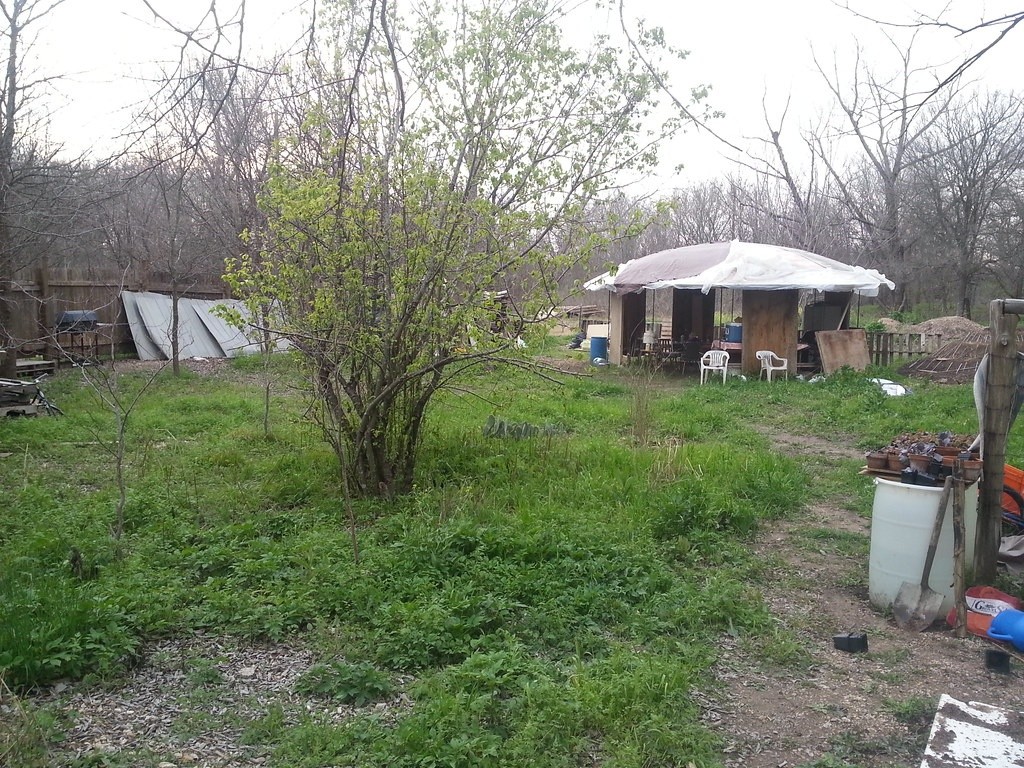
left=660, top=338, right=699, bottom=374
left=700, top=350, right=729, bottom=385
left=754, top=350, right=788, bottom=382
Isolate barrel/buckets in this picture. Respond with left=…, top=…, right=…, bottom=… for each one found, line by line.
left=723, top=323, right=742, bottom=343
left=590, top=336, right=607, bottom=364
left=1001, top=463, right=1024, bottom=515
left=866, top=475, right=980, bottom=625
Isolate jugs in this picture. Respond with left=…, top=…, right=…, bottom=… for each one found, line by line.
left=987, top=609, right=1024, bottom=652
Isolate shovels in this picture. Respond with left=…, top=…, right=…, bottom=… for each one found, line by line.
left=889, top=474, right=956, bottom=635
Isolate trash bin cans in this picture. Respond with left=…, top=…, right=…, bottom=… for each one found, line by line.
left=590, top=337, right=608, bottom=366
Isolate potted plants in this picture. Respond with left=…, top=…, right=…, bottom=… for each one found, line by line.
left=866, top=430, right=986, bottom=483
